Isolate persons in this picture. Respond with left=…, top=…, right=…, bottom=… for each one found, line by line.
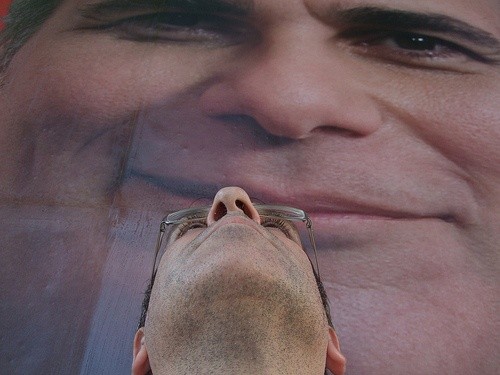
left=0, top=1, right=499, bottom=375
left=132, top=187, right=347, bottom=375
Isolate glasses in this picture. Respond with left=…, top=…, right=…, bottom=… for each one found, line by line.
left=151, top=205, right=321, bottom=288
